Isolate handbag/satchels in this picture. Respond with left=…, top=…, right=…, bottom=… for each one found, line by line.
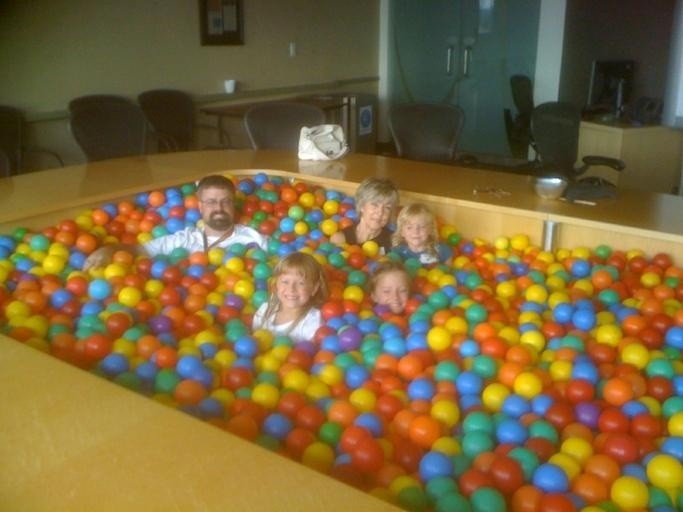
left=298, top=124, right=350, bottom=161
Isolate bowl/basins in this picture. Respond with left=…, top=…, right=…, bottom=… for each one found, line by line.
left=532, top=175, right=568, bottom=201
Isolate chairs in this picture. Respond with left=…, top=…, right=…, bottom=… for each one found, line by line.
left=242, top=102, right=327, bottom=150
left=0, top=90, right=216, bottom=178
left=527, top=96, right=625, bottom=191
left=387, top=100, right=466, bottom=164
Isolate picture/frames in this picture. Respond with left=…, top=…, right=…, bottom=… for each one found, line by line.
left=198, top=0, right=244, bottom=46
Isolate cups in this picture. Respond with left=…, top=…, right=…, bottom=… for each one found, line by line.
left=223, top=80, right=234, bottom=93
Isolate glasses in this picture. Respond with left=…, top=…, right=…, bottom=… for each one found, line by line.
left=199, top=197, right=233, bottom=206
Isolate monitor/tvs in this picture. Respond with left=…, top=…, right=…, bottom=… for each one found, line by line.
left=586, top=59, right=635, bottom=122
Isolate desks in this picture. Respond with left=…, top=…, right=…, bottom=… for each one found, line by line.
left=199, top=94, right=349, bottom=150
left=578, top=119, right=683, bottom=195
